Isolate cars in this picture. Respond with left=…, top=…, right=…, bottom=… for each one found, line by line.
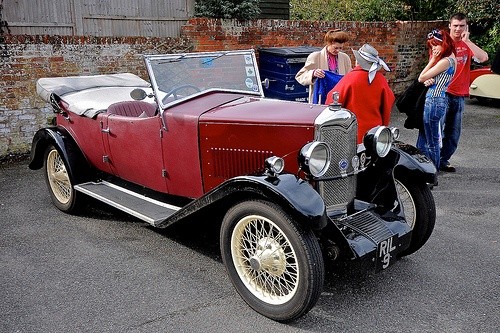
left=28, top=48, right=439, bottom=322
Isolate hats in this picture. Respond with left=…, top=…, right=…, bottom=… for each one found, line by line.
left=352, top=43, right=391, bottom=83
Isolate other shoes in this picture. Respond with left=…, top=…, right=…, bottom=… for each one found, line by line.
left=439, top=164, right=455, bottom=171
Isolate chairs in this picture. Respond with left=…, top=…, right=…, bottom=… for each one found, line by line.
left=106, top=100, right=157, bottom=118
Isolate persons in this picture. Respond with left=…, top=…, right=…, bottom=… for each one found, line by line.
left=295, top=29, right=352, bottom=105
left=416, top=29, right=457, bottom=189
left=429, top=13, right=489, bottom=172
left=325, top=44, right=395, bottom=144
